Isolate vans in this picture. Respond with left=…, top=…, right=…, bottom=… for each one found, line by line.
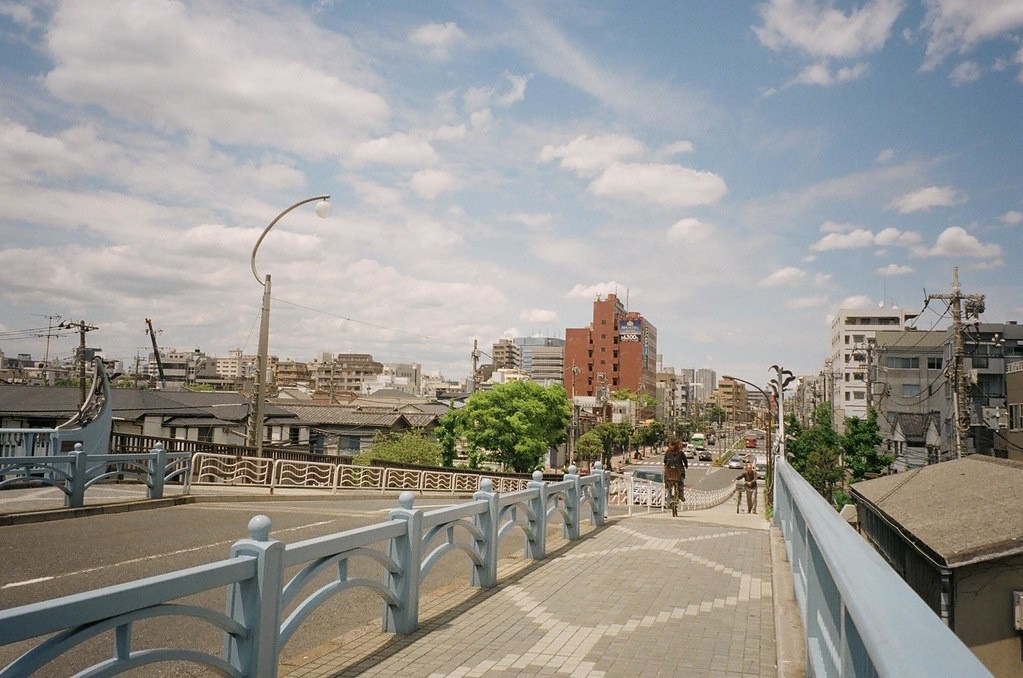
left=751, top=454, right=767, bottom=480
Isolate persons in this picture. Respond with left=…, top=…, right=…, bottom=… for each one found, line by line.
left=664, top=436, right=688, bottom=502
left=732, top=464, right=758, bottom=514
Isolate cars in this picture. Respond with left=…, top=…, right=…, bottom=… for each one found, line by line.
left=626, top=468, right=665, bottom=509
left=698, top=451, right=712, bottom=461
left=681, top=422, right=744, bottom=459
left=734, top=450, right=748, bottom=462
left=728, top=457, right=744, bottom=470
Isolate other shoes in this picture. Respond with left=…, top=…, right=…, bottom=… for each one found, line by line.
left=678, top=492, right=686, bottom=502
left=747, top=512, right=752, bottom=514
left=753, top=511, right=757, bottom=514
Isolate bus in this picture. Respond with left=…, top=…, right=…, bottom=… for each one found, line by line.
left=745, top=434, right=757, bottom=448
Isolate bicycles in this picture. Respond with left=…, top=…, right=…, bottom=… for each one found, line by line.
left=667, top=466, right=683, bottom=517
left=733, top=480, right=748, bottom=514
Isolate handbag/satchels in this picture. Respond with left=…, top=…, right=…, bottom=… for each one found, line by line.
left=667, top=468, right=679, bottom=481
left=736, top=484, right=743, bottom=489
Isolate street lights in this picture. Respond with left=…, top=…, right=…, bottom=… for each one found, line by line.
left=722, top=374, right=771, bottom=486
left=737, top=410, right=769, bottom=478
left=253, top=194, right=333, bottom=489
